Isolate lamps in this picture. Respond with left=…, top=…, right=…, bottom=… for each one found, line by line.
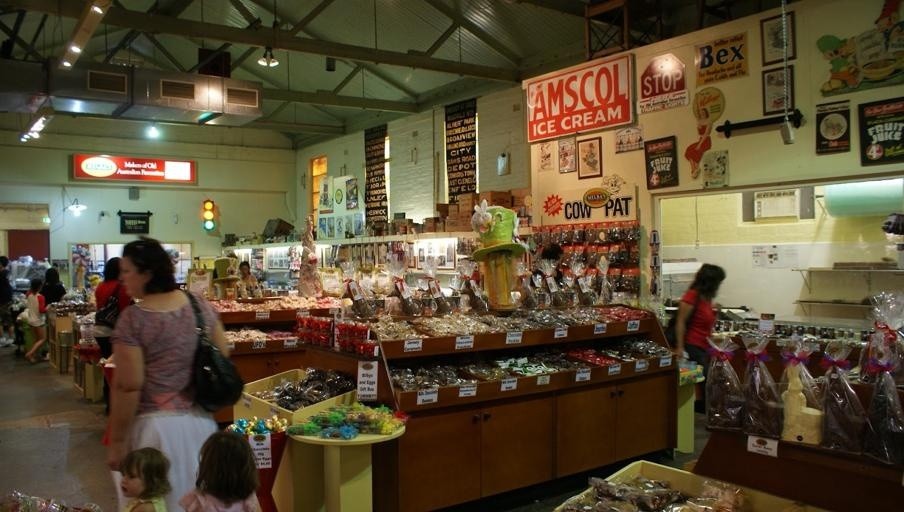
left=19, top=2, right=279, bottom=143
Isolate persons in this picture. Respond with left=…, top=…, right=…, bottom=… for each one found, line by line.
left=0, top=256, right=132, bottom=417
left=528, top=243, right=562, bottom=286
left=238, top=260, right=257, bottom=283
left=106, top=235, right=231, bottom=512
left=683, top=108, right=713, bottom=180
left=108, top=449, right=174, bottom=511
left=177, top=430, right=263, bottom=512
left=673, top=262, right=726, bottom=399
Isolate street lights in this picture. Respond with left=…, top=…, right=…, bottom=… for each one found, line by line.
left=665, top=295, right=700, bottom=349
left=193, top=334, right=243, bottom=412
left=94, top=296, right=120, bottom=329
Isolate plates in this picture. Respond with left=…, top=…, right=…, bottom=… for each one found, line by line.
left=791, top=267, right=904, bottom=324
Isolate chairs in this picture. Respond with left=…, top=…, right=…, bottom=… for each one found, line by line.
left=26, top=352, right=35, bottom=363
left=0, top=337, right=16, bottom=347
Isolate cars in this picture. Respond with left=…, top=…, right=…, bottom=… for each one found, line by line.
left=896, top=244, right=904, bottom=270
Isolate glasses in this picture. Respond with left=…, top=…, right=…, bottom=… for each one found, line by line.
left=19, top=2, right=279, bottom=143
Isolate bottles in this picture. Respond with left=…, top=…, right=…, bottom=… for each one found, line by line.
left=470, top=199, right=526, bottom=260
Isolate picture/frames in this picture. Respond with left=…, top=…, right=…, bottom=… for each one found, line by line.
left=760, top=10, right=798, bottom=66
left=577, top=136, right=602, bottom=179
left=644, top=135, right=679, bottom=190
left=761, top=64, right=795, bottom=115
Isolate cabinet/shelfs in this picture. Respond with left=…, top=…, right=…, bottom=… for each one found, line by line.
left=426, top=250, right=433, bottom=263
left=267, top=255, right=288, bottom=268
left=236, top=233, right=303, bottom=244
left=447, top=244, right=453, bottom=262
left=237, top=282, right=264, bottom=298
left=419, top=246, right=424, bottom=262
left=438, top=252, right=446, bottom=267
left=344, top=223, right=408, bottom=238
left=791, top=267, right=904, bottom=324
left=46, top=295, right=678, bottom=512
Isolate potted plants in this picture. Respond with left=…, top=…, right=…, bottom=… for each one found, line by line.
left=577, top=136, right=602, bottom=179
left=644, top=135, right=679, bottom=190
left=760, top=10, right=798, bottom=66
left=761, top=64, right=795, bottom=115
